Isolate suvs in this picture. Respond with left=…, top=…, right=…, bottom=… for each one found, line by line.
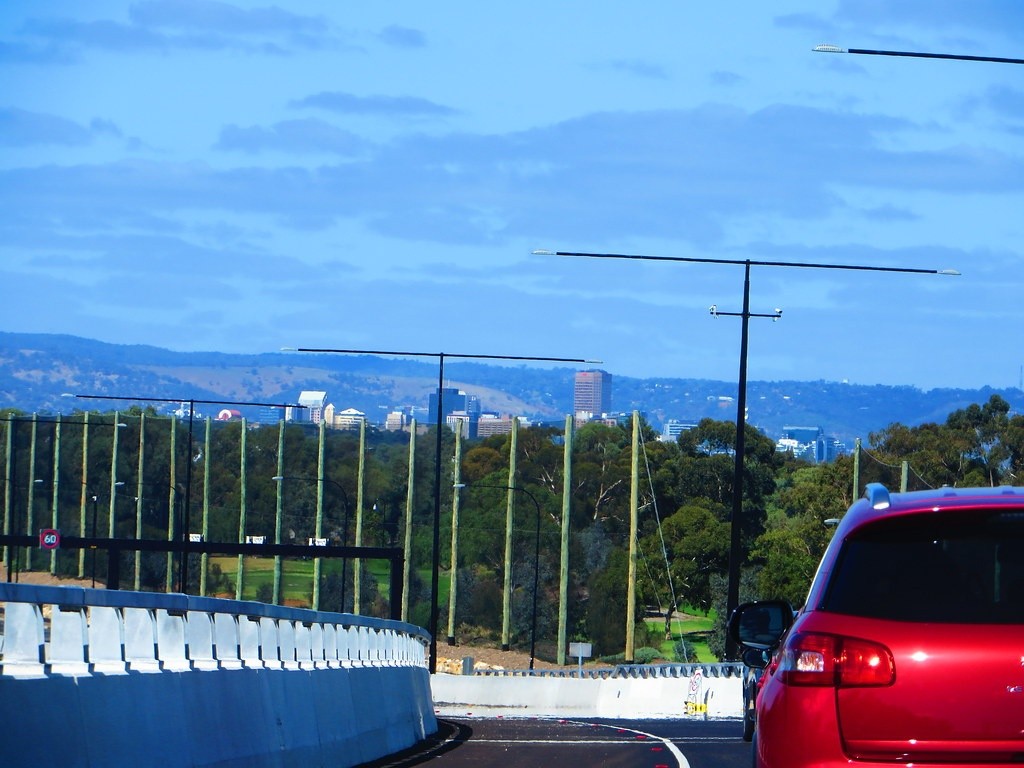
left=725, top=481, right=1024, bottom=766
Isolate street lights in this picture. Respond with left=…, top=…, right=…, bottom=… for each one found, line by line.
left=530, top=251, right=962, bottom=666
left=33, top=476, right=99, bottom=588
left=270, top=476, right=348, bottom=612
left=1, top=419, right=127, bottom=583
left=453, top=481, right=541, bottom=676
left=281, top=347, right=605, bottom=672
left=61, top=393, right=322, bottom=593
left=116, top=481, right=185, bottom=596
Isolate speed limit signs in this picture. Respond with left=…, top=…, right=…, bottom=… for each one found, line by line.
left=39, top=529, right=60, bottom=549
